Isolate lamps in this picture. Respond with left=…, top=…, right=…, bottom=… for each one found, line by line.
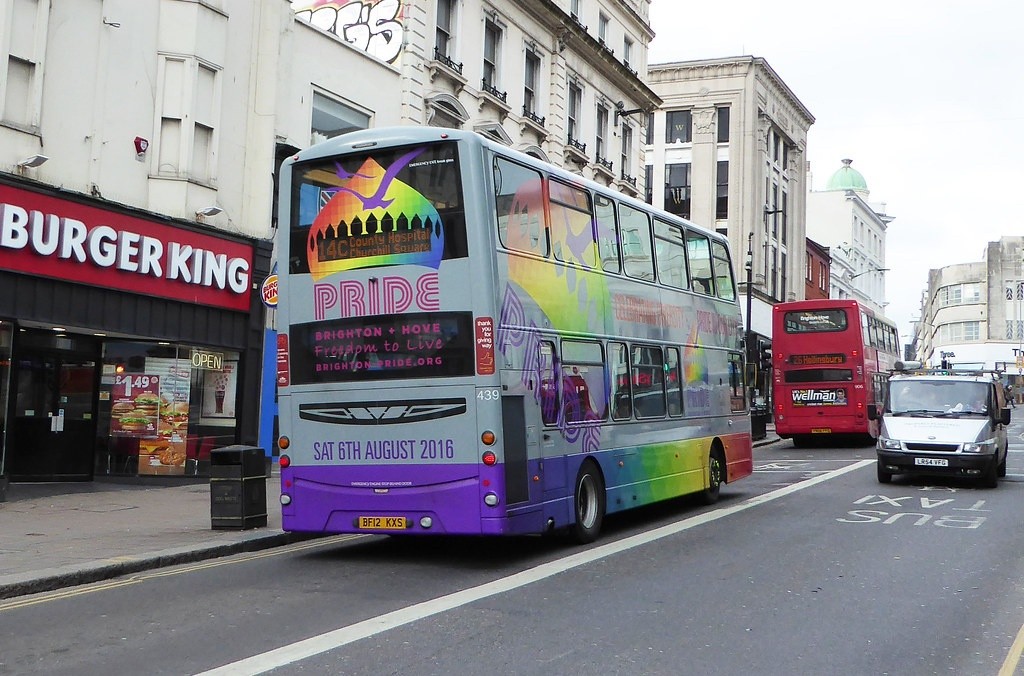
left=195, top=206, right=223, bottom=216
left=17, top=155, right=49, bottom=168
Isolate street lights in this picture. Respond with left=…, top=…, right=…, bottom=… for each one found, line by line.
left=738, top=232, right=764, bottom=331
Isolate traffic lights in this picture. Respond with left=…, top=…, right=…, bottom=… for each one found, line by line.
left=759, top=340, right=771, bottom=370
left=941, top=360, right=947, bottom=375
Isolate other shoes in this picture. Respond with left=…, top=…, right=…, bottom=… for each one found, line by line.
left=1014, top=407, right=1016, bottom=408
left=1004, top=406, right=1007, bottom=407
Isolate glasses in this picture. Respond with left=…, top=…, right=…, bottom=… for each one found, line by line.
left=1010, top=387, right=1012, bottom=388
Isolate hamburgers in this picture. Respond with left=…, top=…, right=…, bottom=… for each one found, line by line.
left=111, top=393, right=189, bottom=455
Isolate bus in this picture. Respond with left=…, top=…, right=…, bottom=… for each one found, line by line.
left=278, top=125, right=754, bottom=544
left=772, top=299, right=901, bottom=447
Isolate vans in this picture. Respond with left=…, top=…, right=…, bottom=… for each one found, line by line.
left=867, top=360, right=1012, bottom=488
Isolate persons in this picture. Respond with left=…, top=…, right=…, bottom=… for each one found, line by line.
left=1004, top=380, right=1016, bottom=409
left=615, top=373, right=638, bottom=418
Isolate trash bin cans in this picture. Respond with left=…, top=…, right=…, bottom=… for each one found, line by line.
left=208, top=444, right=271, bottom=532
left=109, top=435, right=141, bottom=477
left=750, top=404, right=767, bottom=440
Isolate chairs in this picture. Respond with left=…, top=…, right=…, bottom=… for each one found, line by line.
left=196, top=436, right=217, bottom=475
left=120, top=438, right=139, bottom=474
left=186, top=434, right=198, bottom=476
